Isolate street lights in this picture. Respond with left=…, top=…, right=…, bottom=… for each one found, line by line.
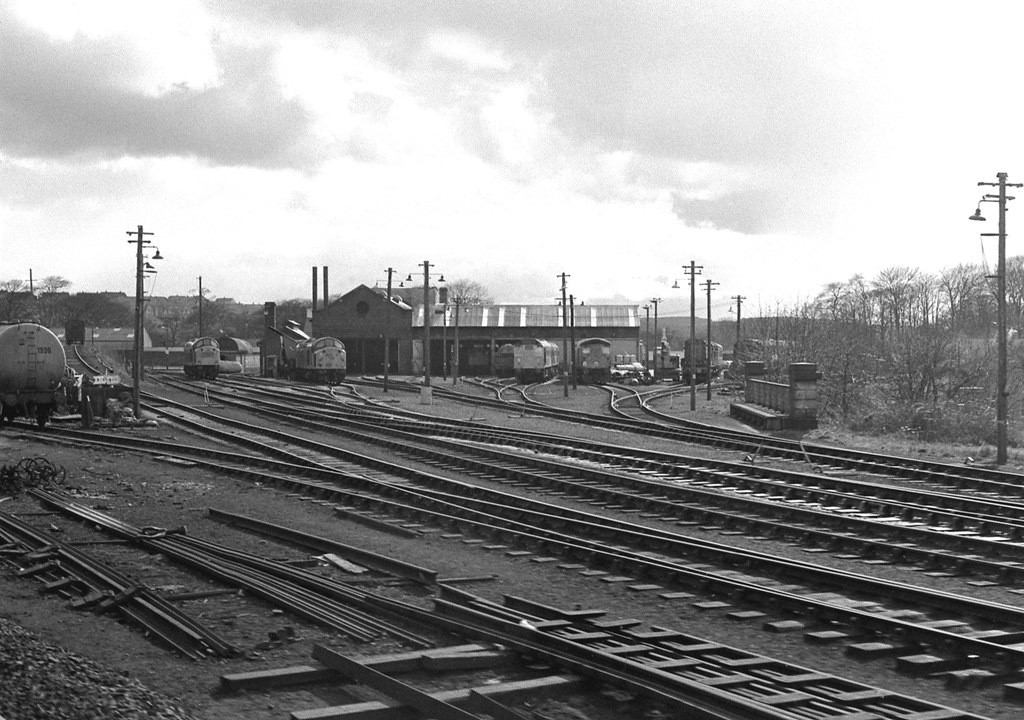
left=133, top=245, right=164, bottom=418
left=373, top=267, right=404, bottom=392
left=970, top=198, right=1008, bottom=462
left=406, top=261, right=445, bottom=387
left=643, top=304, right=653, bottom=385
left=672, top=279, right=696, bottom=412
left=650, top=297, right=663, bottom=383
left=554, top=271, right=585, bottom=397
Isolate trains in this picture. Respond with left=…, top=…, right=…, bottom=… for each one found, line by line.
left=291, top=336, right=346, bottom=385
left=681, top=338, right=723, bottom=383
left=513, top=340, right=558, bottom=385
left=0, top=321, right=66, bottom=428
left=574, top=337, right=612, bottom=387
left=65, top=319, right=85, bottom=344
left=184, top=337, right=219, bottom=379
